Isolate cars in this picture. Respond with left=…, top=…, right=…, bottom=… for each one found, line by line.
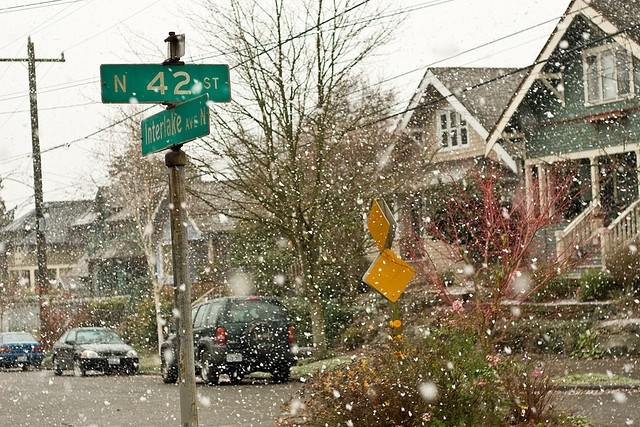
left=51, top=326, right=139, bottom=378
left=0, top=331, right=44, bottom=370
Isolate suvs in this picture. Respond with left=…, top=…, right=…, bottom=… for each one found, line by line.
left=159, top=295, right=297, bottom=385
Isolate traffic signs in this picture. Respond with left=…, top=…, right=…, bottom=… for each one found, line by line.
left=138, top=92, right=211, bottom=157
left=100, top=61, right=232, bottom=104
